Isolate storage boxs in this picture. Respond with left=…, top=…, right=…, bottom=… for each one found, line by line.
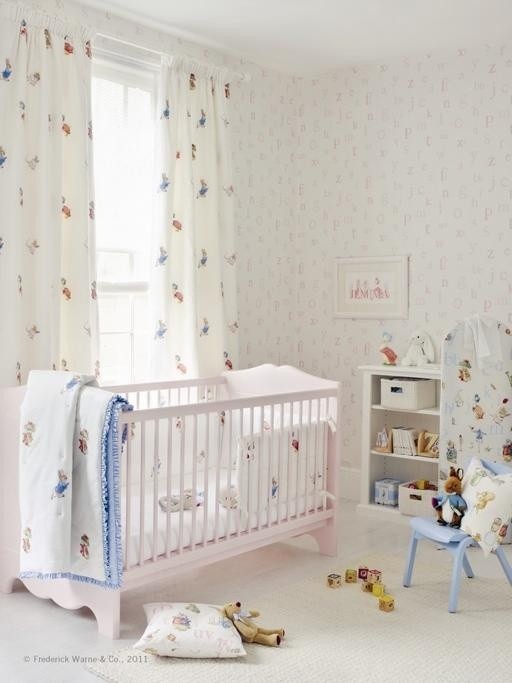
left=372, top=475, right=440, bottom=517
left=377, top=372, right=435, bottom=410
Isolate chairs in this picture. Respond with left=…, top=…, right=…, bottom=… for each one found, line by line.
left=404, top=454, right=512, bottom=612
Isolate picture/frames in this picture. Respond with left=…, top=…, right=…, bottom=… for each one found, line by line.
left=333, top=254, right=411, bottom=320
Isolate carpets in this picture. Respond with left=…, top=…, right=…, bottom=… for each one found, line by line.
left=76, top=548, right=509, bottom=683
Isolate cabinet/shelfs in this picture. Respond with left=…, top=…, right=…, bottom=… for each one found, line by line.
left=358, top=359, right=443, bottom=524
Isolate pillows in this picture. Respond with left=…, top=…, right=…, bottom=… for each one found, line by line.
left=134, top=599, right=246, bottom=660
left=448, top=459, right=512, bottom=556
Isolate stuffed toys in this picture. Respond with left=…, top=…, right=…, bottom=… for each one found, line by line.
left=430, top=466, right=467, bottom=530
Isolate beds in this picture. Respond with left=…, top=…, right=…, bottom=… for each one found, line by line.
left=1, top=361, right=338, bottom=639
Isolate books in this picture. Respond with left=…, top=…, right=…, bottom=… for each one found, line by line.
left=391, top=425, right=418, bottom=456
